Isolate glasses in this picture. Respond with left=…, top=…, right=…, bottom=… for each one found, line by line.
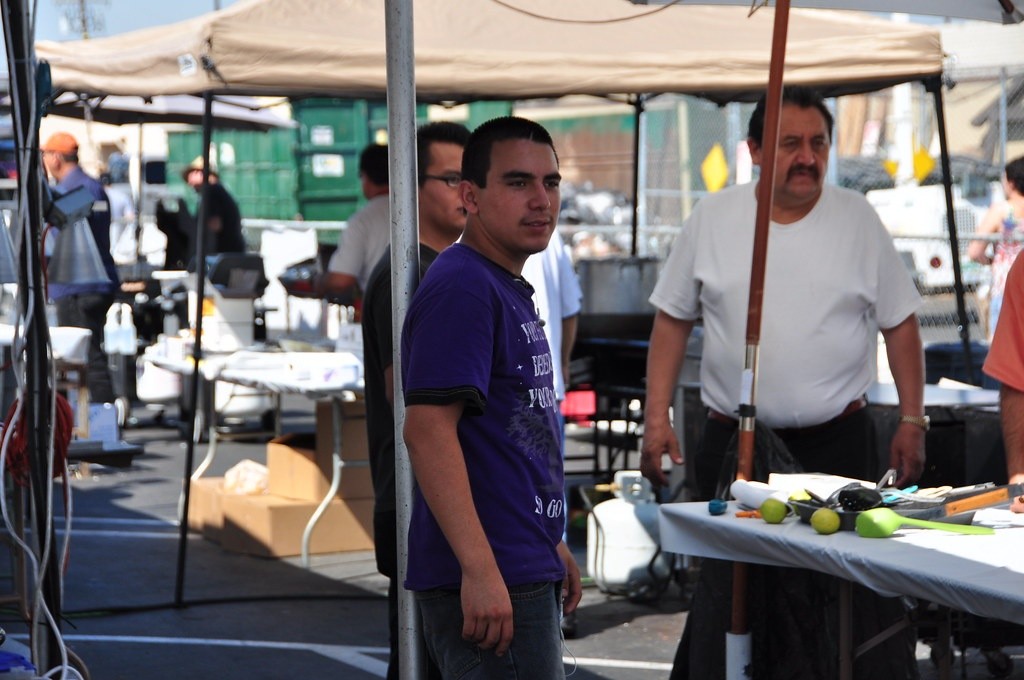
left=424, top=174, right=461, bottom=187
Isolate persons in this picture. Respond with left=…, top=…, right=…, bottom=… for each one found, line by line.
left=180, top=155, right=247, bottom=284
left=36, top=131, right=135, bottom=406
left=402, top=119, right=583, bottom=680
left=363, top=122, right=470, bottom=680
left=639, top=89, right=931, bottom=502
left=326, top=142, right=389, bottom=299
left=521, top=227, right=584, bottom=637
left=980, top=247, right=1024, bottom=514
left=967, top=157, right=1024, bottom=343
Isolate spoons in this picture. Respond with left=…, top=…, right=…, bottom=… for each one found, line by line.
left=856, top=508, right=995, bottom=538
left=839, top=469, right=896, bottom=510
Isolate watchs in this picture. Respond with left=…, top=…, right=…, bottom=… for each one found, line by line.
left=900, top=415, right=931, bottom=431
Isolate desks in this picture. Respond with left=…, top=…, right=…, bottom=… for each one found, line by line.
left=659, top=484, right=1023, bottom=680
left=145, top=350, right=365, bottom=567
left=591, top=338, right=651, bottom=479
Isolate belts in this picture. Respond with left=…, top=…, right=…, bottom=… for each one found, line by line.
left=707, top=393, right=872, bottom=439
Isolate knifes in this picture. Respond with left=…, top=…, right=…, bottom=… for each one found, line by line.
left=906, top=484, right=1024, bottom=521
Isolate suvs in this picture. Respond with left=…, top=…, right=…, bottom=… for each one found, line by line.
left=566, top=257, right=705, bottom=402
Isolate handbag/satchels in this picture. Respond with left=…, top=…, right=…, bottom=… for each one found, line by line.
left=714, top=419, right=803, bottom=500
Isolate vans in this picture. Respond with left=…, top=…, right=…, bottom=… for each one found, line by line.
left=866, top=186, right=999, bottom=294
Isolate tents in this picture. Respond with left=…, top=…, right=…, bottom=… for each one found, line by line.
left=34, top=0, right=1023, bottom=607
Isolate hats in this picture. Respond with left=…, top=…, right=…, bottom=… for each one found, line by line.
left=38, top=132, right=79, bottom=156
left=183, top=156, right=220, bottom=183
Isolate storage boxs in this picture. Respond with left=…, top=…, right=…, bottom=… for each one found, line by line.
left=180, top=397, right=374, bottom=559
left=179, top=267, right=261, bottom=355
